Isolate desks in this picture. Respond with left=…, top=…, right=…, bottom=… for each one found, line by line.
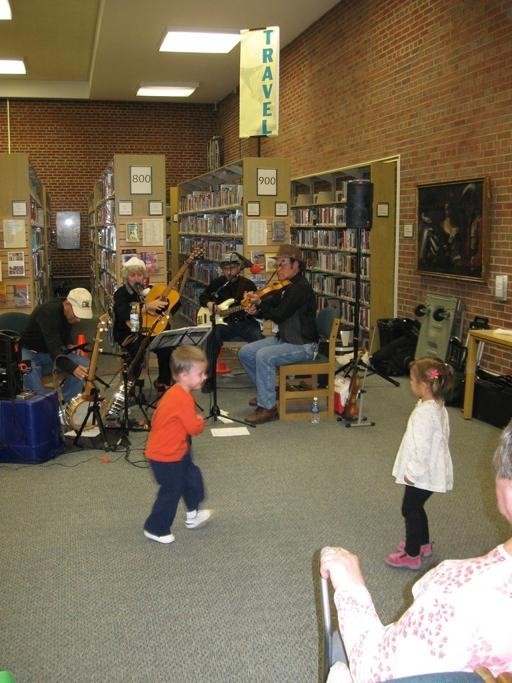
left=463, top=327, right=512, bottom=420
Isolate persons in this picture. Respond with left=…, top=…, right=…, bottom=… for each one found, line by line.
left=112, top=257, right=175, bottom=399
left=19, top=287, right=93, bottom=403
left=198, top=253, right=267, bottom=394
left=141, top=343, right=216, bottom=545
left=318, top=420, right=511, bottom=683
left=384, top=355, right=455, bottom=571
left=236, top=243, right=322, bottom=425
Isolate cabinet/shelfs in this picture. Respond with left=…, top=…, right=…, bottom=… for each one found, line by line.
left=90, top=152, right=171, bottom=328
left=0, top=152, right=53, bottom=316
left=290, top=155, right=401, bottom=369
left=166, top=154, right=294, bottom=335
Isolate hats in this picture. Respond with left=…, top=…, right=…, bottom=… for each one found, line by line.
left=220, top=253, right=240, bottom=266
left=67, top=287, right=94, bottom=319
left=270, top=244, right=303, bottom=264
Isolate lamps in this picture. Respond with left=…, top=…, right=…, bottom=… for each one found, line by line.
left=133, top=82, right=199, bottom=98
left=157, top=25, right=242, bottom=54
left=0, top=57, right=28, bottom=74
left=239, top=24, right=280, bottom=157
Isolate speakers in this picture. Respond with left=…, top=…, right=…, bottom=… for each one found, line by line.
left=345, top=180, right=375, bottom=229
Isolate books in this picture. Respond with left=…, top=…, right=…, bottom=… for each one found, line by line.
left=88, top=160, right=116, bottom=311
left=30, top=199, right=52, bottom=307
left=290, top=206, right=370, bottom=328
left=167, top=184, right=243, bottom=322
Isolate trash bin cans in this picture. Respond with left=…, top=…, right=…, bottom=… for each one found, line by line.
left=378, top=318, right=413, bottom=348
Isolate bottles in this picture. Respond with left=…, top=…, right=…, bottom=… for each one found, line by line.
left=311, top=397, right=320, bottom=424
left=130, top=305, right=139, bottom=332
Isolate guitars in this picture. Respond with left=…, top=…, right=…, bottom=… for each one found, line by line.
left=195, top=298, right=254, bottom=328
left=125, top=244, right=208, bottom=337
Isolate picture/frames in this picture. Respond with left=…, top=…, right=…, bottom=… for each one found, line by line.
left=415, top=177, right=490, bottom=284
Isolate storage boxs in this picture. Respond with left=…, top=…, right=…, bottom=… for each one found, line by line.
left=0, top=389, right=66, bottom=465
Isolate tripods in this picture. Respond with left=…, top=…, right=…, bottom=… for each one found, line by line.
left=334, top=230, right=402, bottom=387
left=202, top=265, right=259, bottom=429
left=73, top=400, right=111, bottom=451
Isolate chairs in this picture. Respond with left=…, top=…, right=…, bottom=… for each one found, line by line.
left=278, top=306, right=340, bottom=422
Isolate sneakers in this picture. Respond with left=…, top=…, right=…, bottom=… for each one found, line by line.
left=398, top=541, right=433, bottom=556
left=384, top=553, right=420, bottom=570
left=249, top=396, right=256, bottom=405
left=247, top=407, right=279, bottom=425
left=144, top=532, right=174, bottom=545
left=185, top=511, right=208, bottom=529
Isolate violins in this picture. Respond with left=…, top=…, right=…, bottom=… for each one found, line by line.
left=240, top=280, right=293, bottom=307
left=343, top=346, right=366, bottom=420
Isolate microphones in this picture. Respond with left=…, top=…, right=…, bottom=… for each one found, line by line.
left=237, top=251, right=254, bottom=266
left=134, top=281, right=141, bottom=290
